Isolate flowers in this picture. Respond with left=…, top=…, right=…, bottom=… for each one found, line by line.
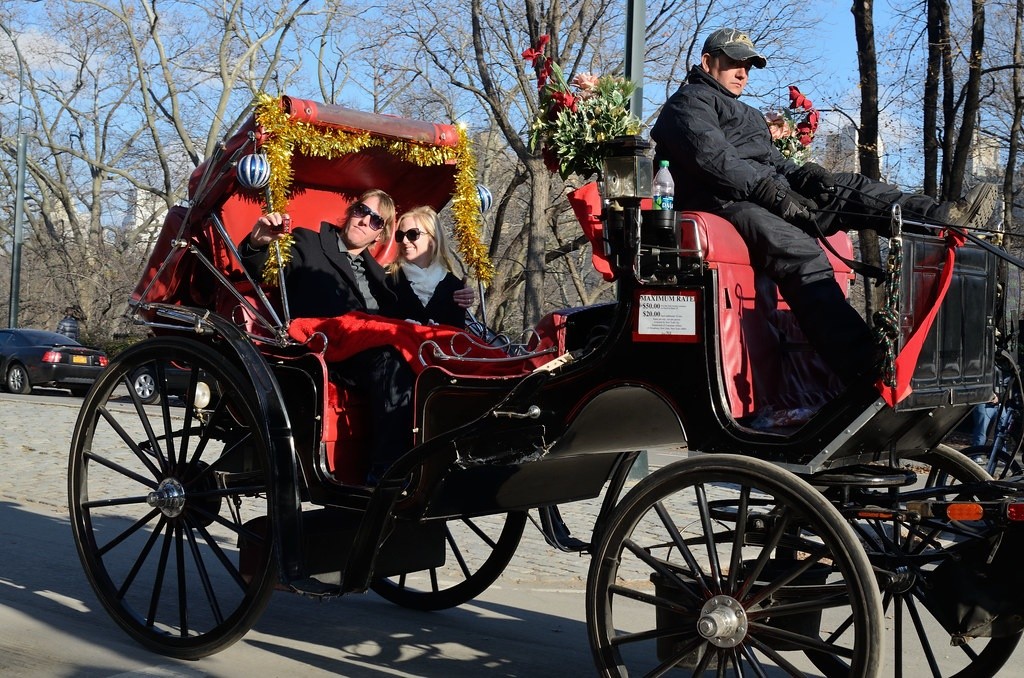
left=517, top=21, right=647, bottom=180
left=764, top=83, right=821, bottom=166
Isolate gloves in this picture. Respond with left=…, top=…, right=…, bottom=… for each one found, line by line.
left=793, top=160, right=837, bottom=205
left=758, top=179, right=819, bottom=225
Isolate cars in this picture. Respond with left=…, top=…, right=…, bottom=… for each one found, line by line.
left=121, top=359, right=220, bottom=405
left=0, top=329, right=108, bottom=397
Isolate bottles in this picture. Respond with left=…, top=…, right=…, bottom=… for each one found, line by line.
left=653, top=160, right=675, bottom=210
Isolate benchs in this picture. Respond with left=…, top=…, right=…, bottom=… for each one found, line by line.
left=675, top=211, right=852, bottom=274
left=232, top=292, right=363, bottom=409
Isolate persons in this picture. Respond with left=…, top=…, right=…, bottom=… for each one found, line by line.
left=386, top=205, right=468, bottom=331
left=649, top=29, right=993, bottom=384
left=237, top=186, right=475, bottom=486
left=972, top=403, right=1013, bottom=466
left=56, top=307, right=80, bottom=344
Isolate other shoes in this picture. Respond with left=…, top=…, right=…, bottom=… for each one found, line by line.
left=374, top=459, right=407, bottom=487
left=932, top=181, right=1000, bottom=237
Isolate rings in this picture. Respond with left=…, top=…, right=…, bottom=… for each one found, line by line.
left=468, top=299, right=470, bottom=304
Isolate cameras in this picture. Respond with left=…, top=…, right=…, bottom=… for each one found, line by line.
left=269, top=218, right=291, bottom=235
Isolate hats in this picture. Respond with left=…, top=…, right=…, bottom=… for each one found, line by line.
left=701, top=28, right=768, bottom=70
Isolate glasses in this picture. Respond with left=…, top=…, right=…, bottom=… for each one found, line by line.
left=394, top=228, right=431, bottom=242
left=351, top=201, right=385, bottom=231
